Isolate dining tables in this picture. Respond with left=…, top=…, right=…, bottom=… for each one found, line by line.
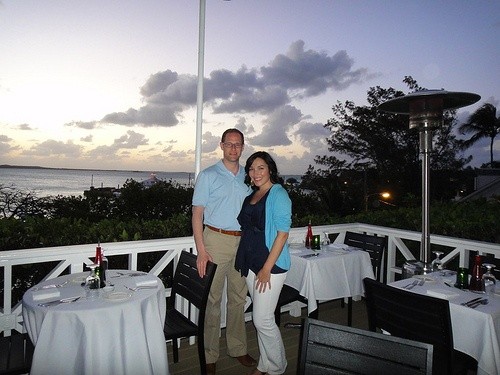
left=0, top=270, right=169, bottom=375
left=382, top=269, right=500, bottom=375
left=284, top=243, right=374, bottom=317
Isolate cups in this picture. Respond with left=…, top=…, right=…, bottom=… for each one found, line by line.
left=456, top=268, right=468, bottom=288
left=312, top=235, right=320, bottom=250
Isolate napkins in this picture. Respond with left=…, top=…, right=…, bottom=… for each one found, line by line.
left=32, top=288, right=60, bottom=301
left=133, top=275, right=157, bottom=287
left=329, top=243, right=349, bottom=248
left=427, top=288, right=459, bottom=300
left=289, top=248, right=301, bottom=253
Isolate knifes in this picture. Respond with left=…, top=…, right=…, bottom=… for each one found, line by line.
left=461, top=297, right=482, bottom=305
left=37, top=296, right=81, bottom=307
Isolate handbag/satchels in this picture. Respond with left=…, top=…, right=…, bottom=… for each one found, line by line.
left=0, top=329, right=35, bottom=375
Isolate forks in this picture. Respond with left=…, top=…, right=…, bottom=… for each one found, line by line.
left=401, top=280, right=425, bottom=290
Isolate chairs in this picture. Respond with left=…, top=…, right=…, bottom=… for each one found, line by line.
left=164, top=231, right=500, bottom=375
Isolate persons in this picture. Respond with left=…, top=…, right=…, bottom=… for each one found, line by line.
left=192, top=129, right=257, bottom=375
left=234, top=151, right=292, bottom=375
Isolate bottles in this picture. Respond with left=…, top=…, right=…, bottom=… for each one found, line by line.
left=470, top=254, right=485, bottom=292
left=306, top=220, right=313, bottom=249
left=94, top=243, right=104, bottom=287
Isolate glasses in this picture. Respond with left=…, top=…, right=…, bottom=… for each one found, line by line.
left=224, top=143, right=243, bottom=148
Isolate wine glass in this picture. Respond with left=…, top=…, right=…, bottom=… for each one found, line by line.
left=85, top=264, right=100, bottom=294
left=321, top=231, right=330, bottom=247
left=481, top=263, right=496, bottom=292
left=431, top=251, right=444, bottom=277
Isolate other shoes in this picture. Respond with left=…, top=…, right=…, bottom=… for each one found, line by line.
left=252, top=369, right=264, bottom=375
left=207, top=363, right=217, bottom=375
left=232, top=354, right=257, bottom=366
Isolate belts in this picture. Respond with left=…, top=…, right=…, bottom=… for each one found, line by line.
left=206, top=225, right=241, bottom=236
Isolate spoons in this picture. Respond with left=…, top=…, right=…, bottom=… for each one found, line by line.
left=471, top=299, right=488, bottom=308
